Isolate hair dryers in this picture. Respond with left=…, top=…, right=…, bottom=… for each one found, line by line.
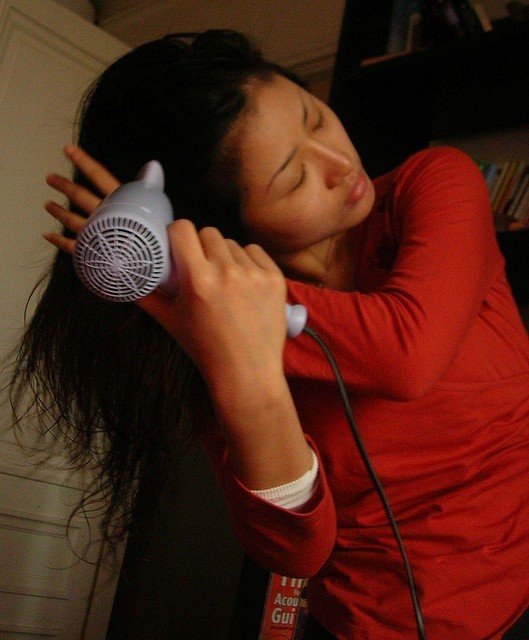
left=72, top=160, right=308, bottom=337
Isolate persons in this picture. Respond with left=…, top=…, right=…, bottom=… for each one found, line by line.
left=13, top=24, right=529, bottom=640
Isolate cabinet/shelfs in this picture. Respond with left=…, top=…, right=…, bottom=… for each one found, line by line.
left=327, top=1, right=529, bottom=329
left=1, top=0, right=136, bottom=632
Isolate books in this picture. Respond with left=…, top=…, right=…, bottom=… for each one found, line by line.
left=428, top=131, right=529, bottom=231
